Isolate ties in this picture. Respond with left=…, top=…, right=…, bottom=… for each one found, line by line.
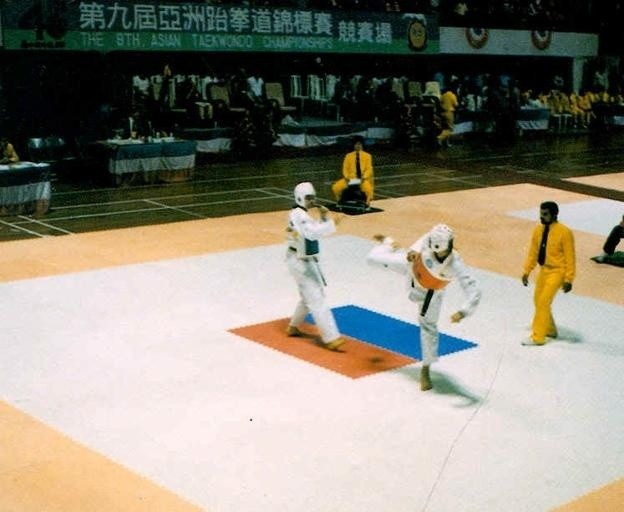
left=356, top=153, right=360, bottom=178
left=539, top=225, right=549, bottom=266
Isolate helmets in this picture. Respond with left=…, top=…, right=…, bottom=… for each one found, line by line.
left=293, top=182, right=316, bottom=208
left=426, top=224, right=454, bottom=252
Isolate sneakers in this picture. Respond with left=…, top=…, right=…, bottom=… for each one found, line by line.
left=523, top=335, right=536, bottom=345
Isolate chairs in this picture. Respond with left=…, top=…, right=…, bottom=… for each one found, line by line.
left=544, top=97, right=584, bottom=129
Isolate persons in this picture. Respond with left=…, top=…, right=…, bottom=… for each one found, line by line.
left=1, top=67, right=266, bottom=165
left=286, top=182, right=345, bottom=350
left=325, top=74, right=623, bottom=149
left=521, top=201, right=577, bottom=346
left=367, top=223, right=482, bottom=391
left=589, top=216, right=624, bottom=267
left=330, top=136, right=374, bottom=211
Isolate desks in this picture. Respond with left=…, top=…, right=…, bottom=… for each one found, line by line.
left=515, top=106, right=551, bottom=137
